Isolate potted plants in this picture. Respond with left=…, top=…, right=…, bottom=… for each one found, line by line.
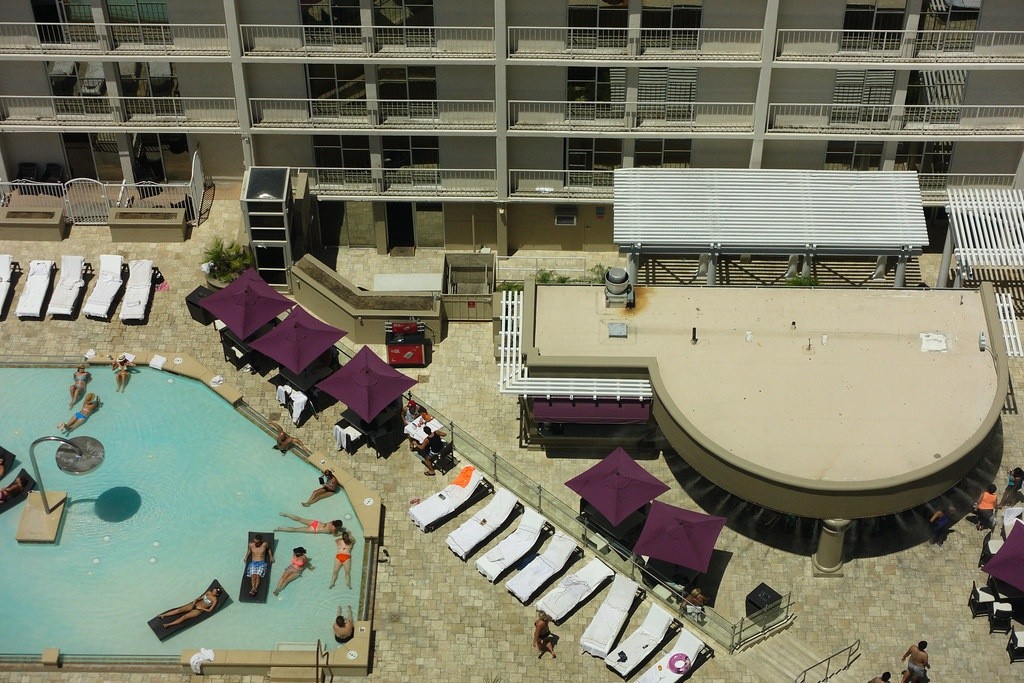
left=199, top=236, right=256, bottom=293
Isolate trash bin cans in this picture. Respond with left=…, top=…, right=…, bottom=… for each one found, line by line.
left=385, top=321, right=433, bottom=366
left=746, top=582, right=783, bottom=622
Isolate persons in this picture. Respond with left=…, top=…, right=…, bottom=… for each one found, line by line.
left=273, top=512, right=352, bottom=536
left=243, top=534, right=275, bottom=594
left=267, top=419, right=307, bottom=455
left=867, top=640, right=931, bottom=683
left=56, top=363, right=99, bottom=433
left=328, top=532, right=356, bottom=590
left=333, top=605, right=355, bottom=643
left=930, top=500, right=948, bottom=544
left=412, top=426, right=447, bottom=476
left=158, top=587, right=222, bottom=628
left=974, top=484, right=998, bottom=530
left=532, top=609, right=556, bottom=659
left=274, top=546, right=316, bottom=595
left=0, top=476, right=27, bottom=505
left=683, top=588, right=705, bottom=607
left=112, top=355, right=136, bottom=393
left=0, top=458, right=5, bottom=477
left=301, top=470, right=341, bottom=507
left=402, top=399, right=420, bottom=451
left=997, top=467, right=1024, bottom=510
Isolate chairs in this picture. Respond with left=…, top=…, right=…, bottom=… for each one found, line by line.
left=1006, top=625, right=1024, bottom=664
left=16, top=163, right=72, bottom=198
left=220, top=341, right=245, bottom=371
left=239, top=532, right=274, bottom=603
left=132, top=164, right=163, bottom=200
left=968, top=581, right=995, bottom=618
left=82, top=62, right=105, bottom=95
left=148, top=579, right=228, bottom=641
left=276, top=383, right=328, bottom=426
left=409, top=440, right=716, bottom=683
left=0, top=259, right=163, bottom=323
left=51, top=62, right=76, bottom=81
left=334, top=424, right=363, bottom=453
left=978, top=531, right=1004, bottom=568
left=988, top=602, right=1013, bottom=635
left=367, top=427, right=400, bottom=458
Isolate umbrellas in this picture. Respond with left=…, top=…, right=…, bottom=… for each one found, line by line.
left=564, top=445, right=728, bottom=574
left=981, top=518, right=1024, bottom=592
left=196, top=265, right=419, bottom=425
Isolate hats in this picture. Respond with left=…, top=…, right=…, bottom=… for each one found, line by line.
left=407, top=400, right=416, bottom=407
left=76, top=364, right=86, bottom=369
left=117, top=354, right=126, bottom=362
left=84, top=393, right=95, bottom=403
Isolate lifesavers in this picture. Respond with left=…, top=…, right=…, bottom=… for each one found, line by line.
left=669, top=653, right=691, bottom=674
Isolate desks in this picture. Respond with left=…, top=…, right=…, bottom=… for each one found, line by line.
left=186, top=286, right=215, bottom=326
left=403, top=415, right=444, bottom=445
left=586, top=508, right=646, bottom=543
left=224, top=323, right=274, bottom=354
left=647, top=558, right=700, bottom=589
left=341, top=408, right=397, bottom=447
left=280, top=366, right=334, bottom=391
left=990, top=575, right=1024, bottom=606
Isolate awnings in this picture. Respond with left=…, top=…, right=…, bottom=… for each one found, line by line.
left=533, top=400, right=651, bottom=420
